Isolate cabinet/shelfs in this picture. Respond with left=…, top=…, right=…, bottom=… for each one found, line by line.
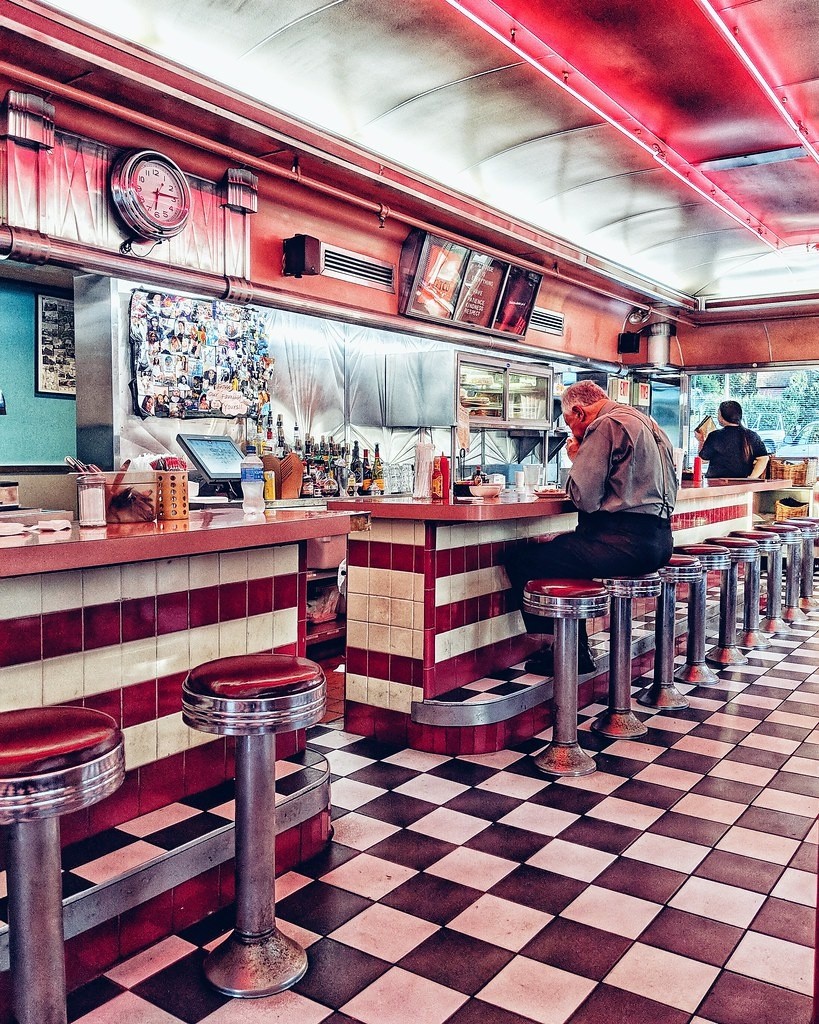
left=307, top=572, right=347, bottom=645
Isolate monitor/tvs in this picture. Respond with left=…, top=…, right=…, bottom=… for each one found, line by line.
left=177, top=434, right=244, bottom=485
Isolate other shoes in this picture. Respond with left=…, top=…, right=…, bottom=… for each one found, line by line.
left=525, top=643, right=598, bottom=677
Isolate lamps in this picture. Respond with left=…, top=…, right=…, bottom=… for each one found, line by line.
left=629, top=309, right=644, bottom=325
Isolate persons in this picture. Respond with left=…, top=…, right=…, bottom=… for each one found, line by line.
left=132, top=289, right=274, bottom=416
left=699, top=400, right=770, bottom=478
left=515, top=379, right=676, bottom=679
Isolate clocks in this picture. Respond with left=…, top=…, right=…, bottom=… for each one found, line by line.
left=106, top=149, right=192, bottom=243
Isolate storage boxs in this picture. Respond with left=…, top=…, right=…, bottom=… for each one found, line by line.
left=68, top=470, right=166, bottom=523
left=306, top=534, right=346, bottom=570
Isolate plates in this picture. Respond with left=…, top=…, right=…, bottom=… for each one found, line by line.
left=456, top=496, right=484, bottom=501
left=533, top=492, right=566, bottom=497
left=460, top=382, right=541, bottom=418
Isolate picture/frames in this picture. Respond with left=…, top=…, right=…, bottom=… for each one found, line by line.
left=34, top=291, right=77, bottom=400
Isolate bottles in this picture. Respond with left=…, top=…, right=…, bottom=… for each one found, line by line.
left=240, top=445, right=265, bottom=515
left=693, top=457, right=702, bottom=481
left=482, top=473, right=489, bottom=483
left=75, top=475, right=109, bottom=527
left=674, top=464, right=679, bottom=490
left=432, top=457, right=444, bottom=499
left=440, top=451, right=449, bottom=499
left=235, top=410, right=385, bottom=498
left=474, top=466, right=482, bottom=486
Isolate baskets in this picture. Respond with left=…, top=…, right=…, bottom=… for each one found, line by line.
left=774, top=500, right=809, bottom=521
left=770, top=455, right=808, bottom=486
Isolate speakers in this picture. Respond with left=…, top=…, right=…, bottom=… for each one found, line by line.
left=284, top=235, right=320, bottom=278
left=619, top=332, right=641, bottom=353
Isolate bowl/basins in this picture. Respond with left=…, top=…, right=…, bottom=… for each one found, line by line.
left=482, top=484, right=504, bottom=493
left=469, top=486, right=500, bottom=497
left=84, top=470, right=166, bottom=523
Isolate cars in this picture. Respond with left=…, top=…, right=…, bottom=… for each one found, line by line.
left=689, top=410, right=819, bottom=473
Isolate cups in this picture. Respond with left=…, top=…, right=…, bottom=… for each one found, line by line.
left=515, top=471, right=524, bottom=486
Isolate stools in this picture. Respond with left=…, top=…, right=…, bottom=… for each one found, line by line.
left=0, top=707, right=126, bottom=1024
left=177, top=655, right=327, bottom=999
left=522, top=517, right=819, bottom=777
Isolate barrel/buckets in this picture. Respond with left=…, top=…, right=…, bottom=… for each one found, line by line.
left=523, top=462, right=544, bottom=486
left=560, top=467, right=571, bottom=486
